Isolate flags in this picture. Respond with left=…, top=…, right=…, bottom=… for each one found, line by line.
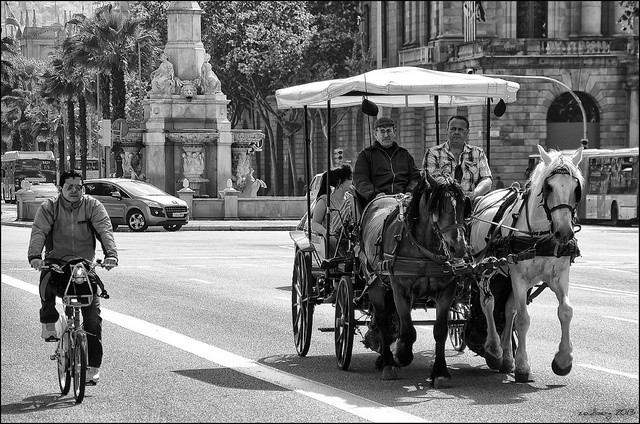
left=473, top=0, right=486, bottom=24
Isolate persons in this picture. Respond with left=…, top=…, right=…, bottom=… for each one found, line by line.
left=311, top=165, right=360, bottom=258
left=495, top=175, right=504, bottom=189
left=424, top=116, right=493, bottom=206
left=298, top=178, right=304, bottom=193
left=27, top=169, right=119, bottom=384
left=353, top=116, right=421, bottom=208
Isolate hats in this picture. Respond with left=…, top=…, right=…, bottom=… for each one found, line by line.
left=373, top=117, right=394, bottom=128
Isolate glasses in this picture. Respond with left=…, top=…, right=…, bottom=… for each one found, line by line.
left=63, top=183, right=83, bottom=191
left=375, top=129, right=394, bottom=136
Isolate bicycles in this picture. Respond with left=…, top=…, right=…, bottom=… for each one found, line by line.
left=30, top=253, right=117, bottom=404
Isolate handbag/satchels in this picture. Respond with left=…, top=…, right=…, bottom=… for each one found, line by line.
left=47, top=257, right=110, bottom=298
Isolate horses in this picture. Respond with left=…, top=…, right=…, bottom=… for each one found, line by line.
left=356, top=164, right=473, bottom=390
left=469, top=143, right=587, bottom=384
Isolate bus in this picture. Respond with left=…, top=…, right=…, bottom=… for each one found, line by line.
left=526, top=146, right=640, bottom=226
left=0, top=150, right=57, bottom=204
left=56, top=155, right=106, bottom=180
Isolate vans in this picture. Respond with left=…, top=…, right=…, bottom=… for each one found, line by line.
left=79, top=176, right=190, bottom=232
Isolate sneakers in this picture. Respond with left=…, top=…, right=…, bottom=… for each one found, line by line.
left=41, top=323, right=57, bottom=339
left=86, top=366, right=100, bottom=383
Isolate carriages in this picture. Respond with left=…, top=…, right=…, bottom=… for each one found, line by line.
left=268, top=64, right=586, bottom=391
